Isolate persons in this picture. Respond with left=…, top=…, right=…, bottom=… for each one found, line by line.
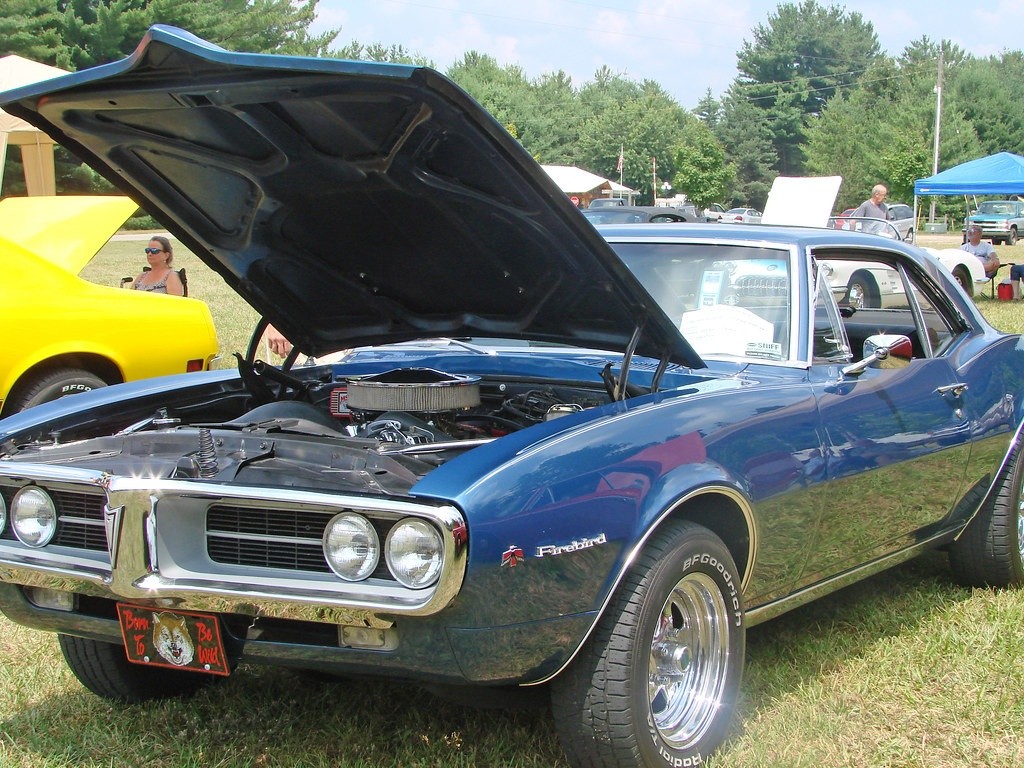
left=959, top=226, right=1000, bottom=278
left=1011, top=264, right=1024, bottom=301
left=130, top=236, right=183, bottom=296
left=268, top=323, right=291, bottom=359
left=849, top=184, right=891, bottom=236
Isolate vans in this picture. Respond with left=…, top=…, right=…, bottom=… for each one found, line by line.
left=843, top=204, right=915, bottom=241
left=587, top=198, right=628, bottom=223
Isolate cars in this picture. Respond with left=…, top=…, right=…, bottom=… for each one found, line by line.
left=580, top=199, right=714, bottom=303
left=1, top=192, right=220, bottom=416
left=828, top=208, right=859, bottom=228
left=719, top=207, right=763, bottom=224
left=1, top=24, right=1024, bottom=768
left=678, top=199, right=725, bottom=222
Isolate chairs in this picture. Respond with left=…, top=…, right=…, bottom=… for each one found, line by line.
left=962, top=242, right=1016, bottom=300
left=120, top=266, right=189, bottom=299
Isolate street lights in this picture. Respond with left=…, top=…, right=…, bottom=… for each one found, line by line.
left=931, top=83, right=942, bottom=222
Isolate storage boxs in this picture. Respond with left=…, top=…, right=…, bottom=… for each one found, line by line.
left=997, top=280, right=1014, bottom=301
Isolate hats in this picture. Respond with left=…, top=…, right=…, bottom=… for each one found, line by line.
left=967, top=226, right=982, bottom=235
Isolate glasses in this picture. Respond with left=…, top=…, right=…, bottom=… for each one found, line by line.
left=145, top=248, right=163, bottom=254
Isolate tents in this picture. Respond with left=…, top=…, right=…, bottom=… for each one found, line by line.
left=912, top=151, right=1024, bottom=247
left=0, top=53, right=75, bottom=199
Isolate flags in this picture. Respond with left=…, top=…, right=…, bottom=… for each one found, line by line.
left=617, top=149, right=624, bottom=171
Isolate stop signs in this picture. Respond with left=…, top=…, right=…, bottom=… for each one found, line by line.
left=570, top=196, right=579, bottom=206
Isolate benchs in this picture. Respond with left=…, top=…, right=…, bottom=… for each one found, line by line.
left=774, top=321, right=939, bottom=366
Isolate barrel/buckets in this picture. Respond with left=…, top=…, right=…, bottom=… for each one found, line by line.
left=997, top=283, right=1012, bottom=300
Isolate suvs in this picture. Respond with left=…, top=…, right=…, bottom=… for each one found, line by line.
left=962, top=200, right=1024, bottom=246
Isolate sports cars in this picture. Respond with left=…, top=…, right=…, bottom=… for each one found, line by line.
left=709, top=174, right=990, bottom=314
